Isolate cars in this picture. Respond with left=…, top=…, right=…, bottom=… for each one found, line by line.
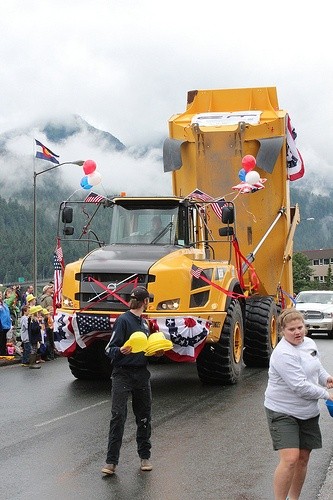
left=295, top=290, right=333, bottom=337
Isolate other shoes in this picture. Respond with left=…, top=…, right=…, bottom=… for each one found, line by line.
left=21, top=362, right=29, bottom=367
left=42, top=356, right=51, bottom=361
left=36, top=359, right=45, bottom=364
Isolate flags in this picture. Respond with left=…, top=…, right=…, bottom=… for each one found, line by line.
left=190, top=265, right=204, bottom=279
left=84, top=191, right=105, bottom=205
left=54, top=242, right=65, bottom=309
left=34, top=138, right=61, bottom=164
left=186, top=189, right=230, bottom=219
left=73, top=313, right=118, bottom=348
left=284, top=114, right=304, bottom=182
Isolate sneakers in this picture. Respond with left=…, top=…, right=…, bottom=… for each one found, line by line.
left=140, top=458, right=152, bottom=471
left=101, top=464, right=116, bottom=475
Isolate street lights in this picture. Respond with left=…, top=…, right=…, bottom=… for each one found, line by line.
left=33, top=160, right=86, bottom=300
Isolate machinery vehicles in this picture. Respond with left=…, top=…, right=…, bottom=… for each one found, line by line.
left=57, top=85, right=305, bottom=387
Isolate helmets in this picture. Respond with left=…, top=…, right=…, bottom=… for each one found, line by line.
left=143, top=332, right=174, bottom=357
left=124, top=331, right=149, bottom=353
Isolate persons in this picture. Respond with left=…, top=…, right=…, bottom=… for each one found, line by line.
left=264, top=307, right=333, bottom=500
left=101, top=287, right=166, bottom=476
left=151, top=217, right=164, bottom=233
left=0, top=282, right=56, bottom=369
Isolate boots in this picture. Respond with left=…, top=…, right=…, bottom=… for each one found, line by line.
left=28, top=354, right=41, bottom=369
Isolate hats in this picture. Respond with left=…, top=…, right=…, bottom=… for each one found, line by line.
left=29, top=306, right=51, bottom=315
left=42, top=285, right=52, bottom=292
left=130, top=286, right=154, bottom=301
left=26, top=294, right=36, bottom=302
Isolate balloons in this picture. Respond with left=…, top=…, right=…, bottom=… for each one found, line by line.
left=239, top=154, right=262, bottom=184
left=84, top=161, right=96, bottom=174
